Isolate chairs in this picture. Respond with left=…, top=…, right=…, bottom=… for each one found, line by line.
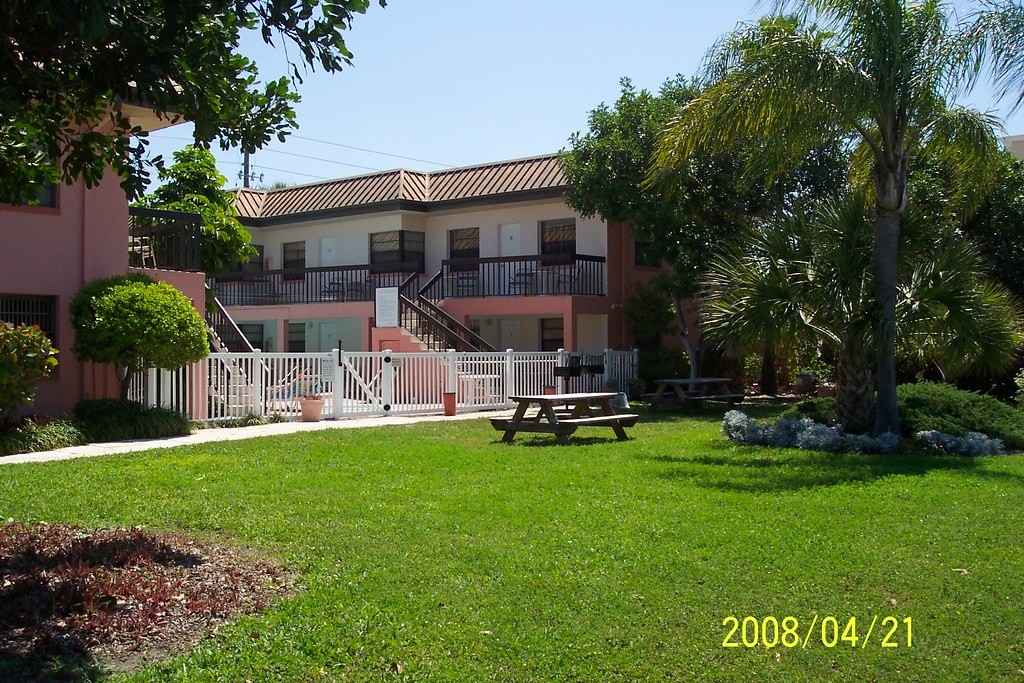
left=322, top=277, right=347, bottom=302
left=507, top=268, right=534, bottom=294
left=456, top=275, right=479, bottom=296
left=347, top=276, right=374, bottom=301
left=555, top=264, right=583, bottom=294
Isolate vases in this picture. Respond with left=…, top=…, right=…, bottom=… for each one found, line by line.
left=544, top=385, right=558, bottom=406
left=443, top=392, right=456, bottom=416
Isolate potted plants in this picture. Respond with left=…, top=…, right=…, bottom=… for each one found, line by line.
left=299, top=392, right=325, bottom=422
left=627, top=378, right=647, bottom=401
left=601, top=378, right=620, bottom=392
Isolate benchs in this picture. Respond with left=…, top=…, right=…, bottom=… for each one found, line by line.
left=490, top=413, right=589, bottom=443
left=641, top=389, right=700, bottom=410
left=688, top=394, right=745, bottom=414
left=557, top=414, right=639, bottom=444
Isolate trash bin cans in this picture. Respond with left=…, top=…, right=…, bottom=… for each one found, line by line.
left=545, top=385, right=558, bottom=407
left=443, top=392, right=457, bottom=416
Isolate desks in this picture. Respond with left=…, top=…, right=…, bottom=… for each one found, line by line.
left=648, top=378, right=737, bottom=416
left=501, top=392, right=628, bottom=446
left=459, top=375, right=501, bottom=402
